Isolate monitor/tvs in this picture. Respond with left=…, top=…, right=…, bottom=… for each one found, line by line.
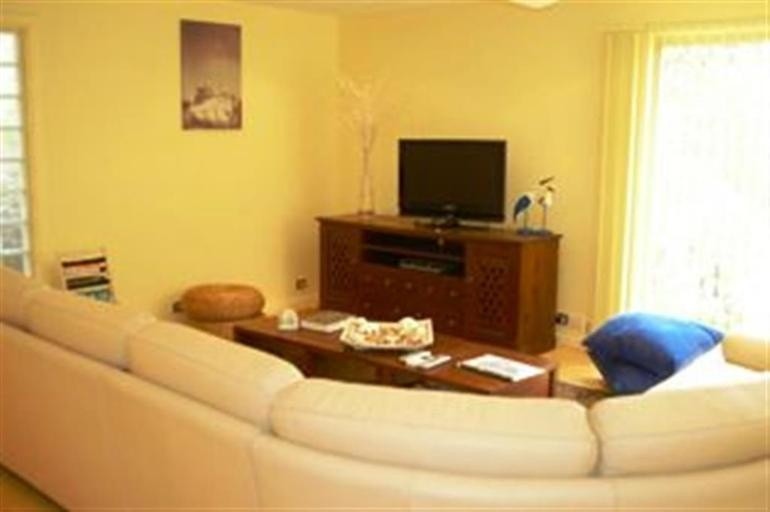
left=397, top=138, right=509, bottom=229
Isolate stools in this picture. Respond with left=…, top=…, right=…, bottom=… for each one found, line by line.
left=171, top=283, right=265, bottom=340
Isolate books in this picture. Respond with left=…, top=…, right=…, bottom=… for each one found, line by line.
left=300, top=309, right=355, bottom=334
left=462, top=352, right=543, bottom=382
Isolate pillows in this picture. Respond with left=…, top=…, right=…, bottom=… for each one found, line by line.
left=585, top=347, right=663, bottom=395
left=580, top=313, right=722, bottom=378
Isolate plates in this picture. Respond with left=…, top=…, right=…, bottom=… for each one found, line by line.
left=340, top=315, right=433, bottom=351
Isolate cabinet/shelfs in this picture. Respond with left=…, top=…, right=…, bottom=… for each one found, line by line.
left=313, top=213, right=563, bottom=355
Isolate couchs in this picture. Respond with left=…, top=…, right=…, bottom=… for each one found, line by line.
left=1, top=266, right=770, bottom=511
